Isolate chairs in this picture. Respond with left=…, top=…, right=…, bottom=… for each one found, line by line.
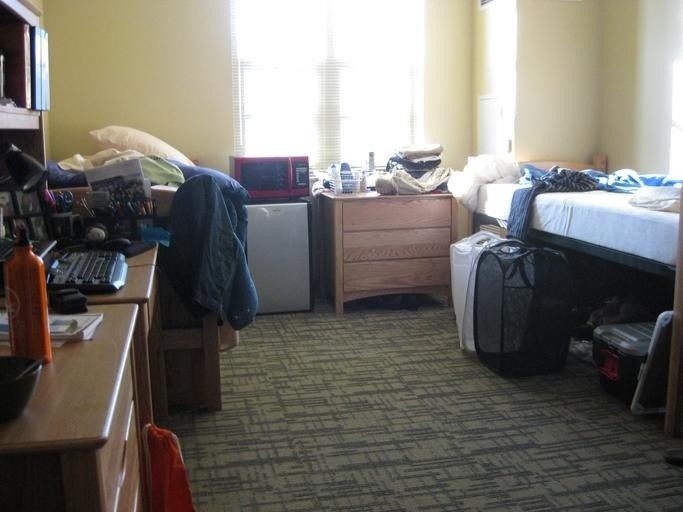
left=151, top=172, right=242, bottom=417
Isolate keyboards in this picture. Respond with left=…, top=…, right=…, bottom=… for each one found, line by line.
left=46, top=250, right=128, bottom=293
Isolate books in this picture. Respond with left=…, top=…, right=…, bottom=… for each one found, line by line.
left=0, top=313, right=103, bottom=342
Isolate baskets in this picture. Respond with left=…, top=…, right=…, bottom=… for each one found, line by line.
left=328, top=169, right=362, bottom=197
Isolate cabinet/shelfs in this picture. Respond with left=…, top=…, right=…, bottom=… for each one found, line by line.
left=318, top=187, right=459, bottom=321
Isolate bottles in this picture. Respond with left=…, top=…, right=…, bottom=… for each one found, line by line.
left=330, top=163, right=354, bottom=195
left=368, top=152, right=376, bottom=171
left=3, top=224, right=52, bottom=363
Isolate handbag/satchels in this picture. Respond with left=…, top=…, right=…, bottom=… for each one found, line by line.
left=140, top=423, right=193, bottom=512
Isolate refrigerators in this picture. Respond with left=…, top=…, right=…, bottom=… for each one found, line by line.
left=241, top=202, right=311, bottom=315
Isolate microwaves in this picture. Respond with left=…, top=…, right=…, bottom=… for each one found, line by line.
left=229, top=156, right=309, bottom=201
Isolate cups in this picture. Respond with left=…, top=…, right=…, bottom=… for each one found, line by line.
left=49, top=211, right=83, bottom=248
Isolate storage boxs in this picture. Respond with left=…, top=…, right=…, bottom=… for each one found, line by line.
left=587, top=319, right=669, bottom=405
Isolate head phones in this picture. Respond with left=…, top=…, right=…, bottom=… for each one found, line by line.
left=86, top=223, right=108, bottom=243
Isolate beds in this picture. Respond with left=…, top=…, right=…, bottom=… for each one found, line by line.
left=448, top=151, right=683, bottom=436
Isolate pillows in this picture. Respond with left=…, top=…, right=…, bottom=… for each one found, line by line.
left=87, top=123, right=253, bottom=201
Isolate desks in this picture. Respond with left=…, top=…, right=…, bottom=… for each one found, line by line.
left=0, top=230, right=170, bottom=511
